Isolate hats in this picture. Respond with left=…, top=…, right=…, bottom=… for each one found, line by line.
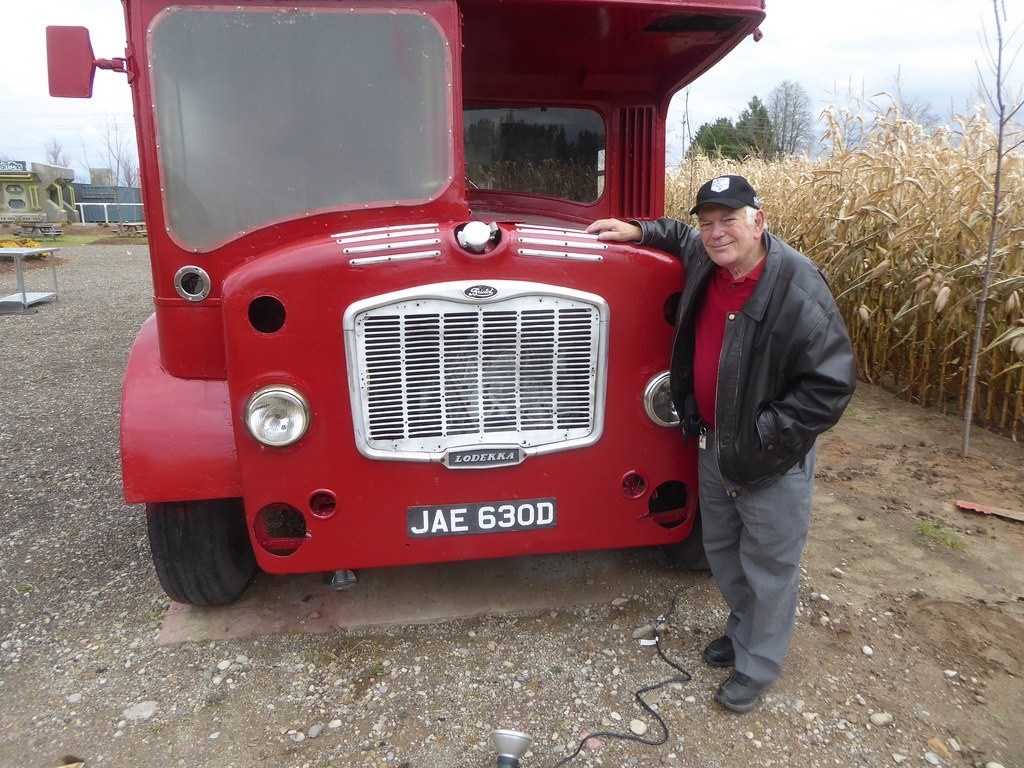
left=690, top=175, right=758, bottom=215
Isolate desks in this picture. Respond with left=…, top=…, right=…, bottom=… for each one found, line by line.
left=21, top=223, right=56, bottom=242
left=117, top=223, right=146, bottom=237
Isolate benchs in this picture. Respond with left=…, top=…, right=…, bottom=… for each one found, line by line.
left=112, top=229, right=127, bottom=232
left=136, top=230, right=148, bottom=233
left=12, top=230, right=64, bottom=235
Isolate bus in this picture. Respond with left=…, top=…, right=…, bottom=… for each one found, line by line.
left=47, top=0, right=770, bottom=609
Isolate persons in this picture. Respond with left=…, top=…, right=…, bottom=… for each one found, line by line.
left=586, top=175, right=857, bottom=712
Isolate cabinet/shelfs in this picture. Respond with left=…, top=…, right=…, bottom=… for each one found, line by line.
left=0, top=247, right=60, bottom=308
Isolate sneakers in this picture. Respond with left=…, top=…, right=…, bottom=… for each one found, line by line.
left=703, top=635, right=736, bottom=667
left=716, top=670, right=762, bottom=711
